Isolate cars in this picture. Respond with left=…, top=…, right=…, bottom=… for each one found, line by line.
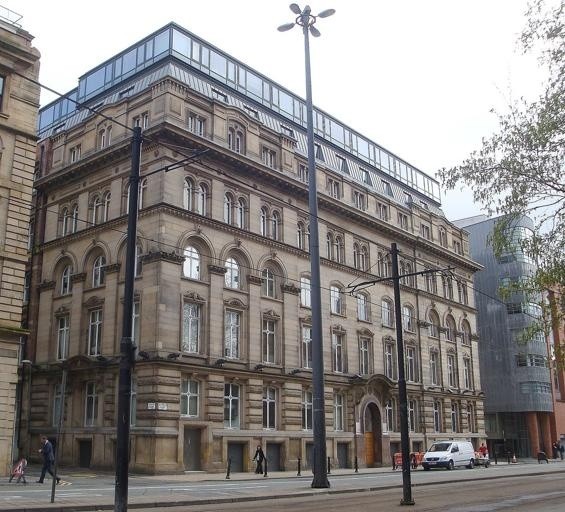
left=475, top=451, right=491, bottom=468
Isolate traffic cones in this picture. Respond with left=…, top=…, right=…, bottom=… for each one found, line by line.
left=512, top=453, right=518, bottom=463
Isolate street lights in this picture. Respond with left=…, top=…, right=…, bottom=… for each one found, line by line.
left=276, top=1, right=338, bottom=489
left=22, top=359, right=69, bottom=503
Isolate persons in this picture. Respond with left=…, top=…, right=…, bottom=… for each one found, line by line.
left=253, top=445, right=265, bottom=474
left=553, top=439, right=563, bottom=459
left=477, top=442, right=487, bottom=457
left=36, top=436, right=61, bottom=485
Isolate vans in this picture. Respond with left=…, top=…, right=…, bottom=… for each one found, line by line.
left=421, top=441, right=475, bottom=470
